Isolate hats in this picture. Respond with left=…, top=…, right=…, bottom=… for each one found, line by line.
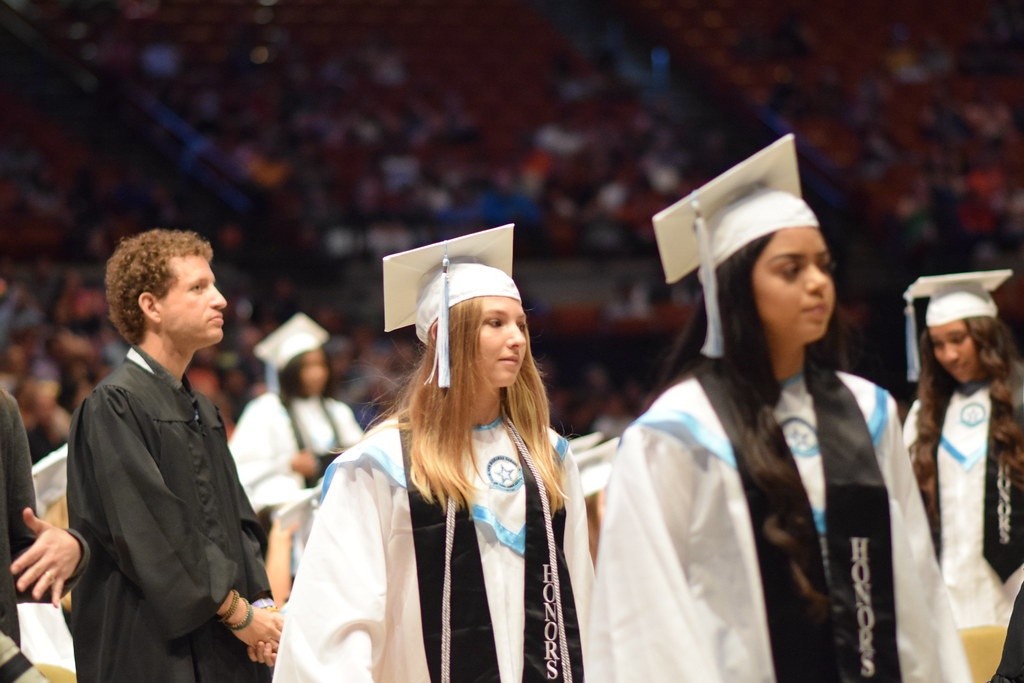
left=902, top=268, right=1013, bottom=383
left=379, top=220, right=523, bottom=389
left=650, top=130, right=821, bottom=360
left=252, top=310, right=332, bottom=394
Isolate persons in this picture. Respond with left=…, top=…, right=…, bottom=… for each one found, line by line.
left=272, top=224, right=594, bottom=683
left=227, top=313, right=365, bottom=513
left=0, top=386, right=90, bottom=683
left=66, top=230, right=285, bottom=683
left=0, top=0, right=1024, bottom=443
left=902, top=269, right=1024, bottom=627
left=569, top=133, right=972, bottom=683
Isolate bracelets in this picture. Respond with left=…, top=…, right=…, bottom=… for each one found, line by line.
left=251, top=597, right=276, bottom=608
left=218, top=589, right=254, bottom=631
